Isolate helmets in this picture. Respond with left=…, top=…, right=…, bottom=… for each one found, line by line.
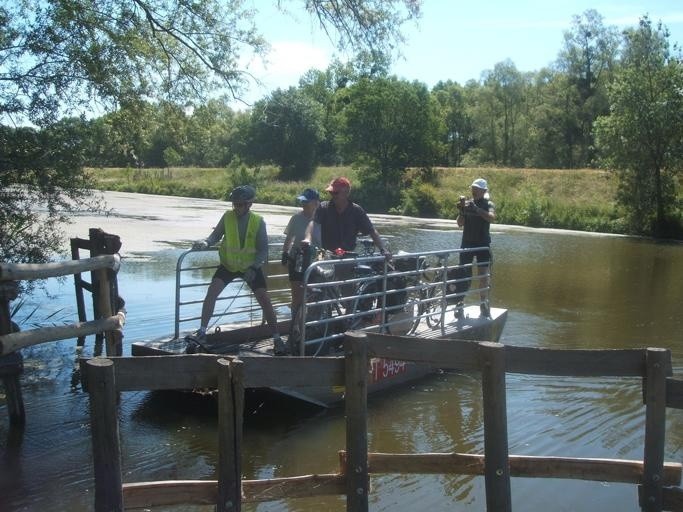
left=230, top=185, right=256, bottom=202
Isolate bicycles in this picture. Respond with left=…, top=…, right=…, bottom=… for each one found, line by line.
left=288, top=240, right=447, bottom=358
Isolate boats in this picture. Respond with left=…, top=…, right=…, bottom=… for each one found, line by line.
left=131, top=294, right=509, bottom=409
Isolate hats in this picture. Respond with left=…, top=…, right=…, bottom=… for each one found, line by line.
left=471, top=178, right=488, bottom=190
left=296, top=189, right=320, bottom=201
left=325, top=177, right=351, bottom=193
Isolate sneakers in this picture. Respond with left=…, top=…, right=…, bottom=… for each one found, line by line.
left=454, top=303, right=464, bottom=317
left=480, top=303, right=490, bottom=316
left=274, top=339, right=286, bottom=354
left=185, top=329, right=208, bottom=345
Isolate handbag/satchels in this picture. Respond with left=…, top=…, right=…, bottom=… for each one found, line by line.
left=446, top=268, right=472, bottom=305
left=377, top=277, right=407, bottom=315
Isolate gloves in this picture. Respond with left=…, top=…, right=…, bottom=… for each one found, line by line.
left=282, top=252, right=288, bottom=265
left=192, top=240, right=210, bottom=251
left=244, top=267, right=257, bottom=283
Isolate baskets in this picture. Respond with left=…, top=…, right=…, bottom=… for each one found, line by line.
left=396, top=259, right=425, bottom=276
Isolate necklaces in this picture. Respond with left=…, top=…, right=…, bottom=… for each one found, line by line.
left=336, top=206, right=344, bottom=210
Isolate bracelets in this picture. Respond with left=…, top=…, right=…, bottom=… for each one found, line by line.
left=249, top=266, right=257, bottom=272
left=205, top=240, right=211, bottom=247
left=458, top=212, right=464, bottom=216
left=380, top=248, right=386, bottom=254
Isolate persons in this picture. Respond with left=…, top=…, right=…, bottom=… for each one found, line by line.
left=453, top=178, right=497, bottom=319
left=281, top=188, right=322, bottom=354
left=184, top=184, right=286, bottom=356
left=309, top=176, right=393, bottom=356
